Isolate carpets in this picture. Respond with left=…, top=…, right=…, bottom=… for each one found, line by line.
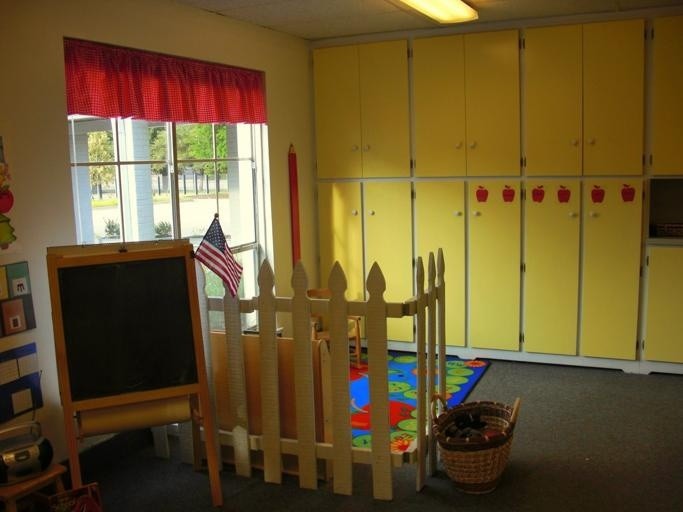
left=348, top=344, right=494, bottom=451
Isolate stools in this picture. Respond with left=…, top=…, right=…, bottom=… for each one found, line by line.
left=1, top=463, right=67, bottom=512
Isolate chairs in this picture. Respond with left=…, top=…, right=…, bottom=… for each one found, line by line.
left=293, top=288, right=364, bottom=367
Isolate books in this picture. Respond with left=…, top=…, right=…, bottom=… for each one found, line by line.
left=48, top=480, right=102, bottom=512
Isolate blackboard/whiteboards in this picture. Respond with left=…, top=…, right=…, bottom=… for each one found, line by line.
left=45, top=244, right=207, bottom=411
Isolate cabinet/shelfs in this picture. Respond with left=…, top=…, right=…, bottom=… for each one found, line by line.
left=311, top=16, right=683, bottom=364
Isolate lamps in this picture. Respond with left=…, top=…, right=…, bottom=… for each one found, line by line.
left=399, top=0, right=478, bottom=27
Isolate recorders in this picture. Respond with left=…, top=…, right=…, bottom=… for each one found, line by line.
left=0, top=421, right=54, bottom=484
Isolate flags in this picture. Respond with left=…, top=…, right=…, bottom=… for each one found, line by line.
left=192, top=218, right=245, bottom=299
